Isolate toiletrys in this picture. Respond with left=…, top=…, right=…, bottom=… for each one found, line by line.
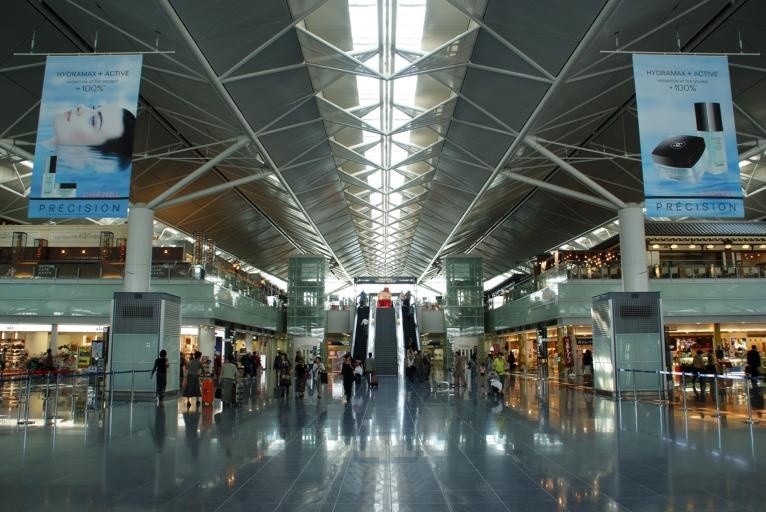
left=651, top=102, right=728, bottom=184
left=41, top=155, right=76, bottom=197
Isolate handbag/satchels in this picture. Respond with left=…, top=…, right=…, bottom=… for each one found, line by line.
left=320, top=373, right=328, bottom=383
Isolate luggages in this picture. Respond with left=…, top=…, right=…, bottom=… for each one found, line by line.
left=371, top=375, right=379, bottom=388
left=202, top=378, right=213, bottom=407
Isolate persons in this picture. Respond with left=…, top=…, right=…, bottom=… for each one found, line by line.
left=150, top=350, right=169, bottom=404
left=406, top=335, right=433, bottom=383
left=747, top=344, right=761, bottom=385
left=361, top=317, right=368, bottom=337
left=0, top=348, right=7, bottom=369
left=46, top=349, right=53, bottom=367
left=692, top=348, right=718, bottom=386
left=452, top=351, right=515, bottom=395
left=582, top=350, right=593, bottom=385
left=180, top=351, right=376, bottom=406
left=400, top=289, right=415, bottom=314
left=356, top=289, right=369, bottom=308
left=21, top=353, right=29, bottom=361
left=41, top=103, right=135, bottom=159
